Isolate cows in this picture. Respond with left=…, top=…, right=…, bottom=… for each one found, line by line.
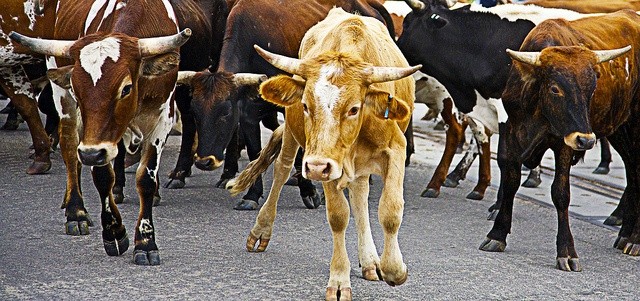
left=396, top=0, right=627, bottom=228
left=224, top=6, right=425, bottom=301
left=0, top=1, right=61, bottom=177
left=178, top=0, right=397, bottom=213
left=8, top=0, right=195, bottom=268
left=479, top=7, right=640, bottom=274
left=163, top=0, right=241, bottom=191
